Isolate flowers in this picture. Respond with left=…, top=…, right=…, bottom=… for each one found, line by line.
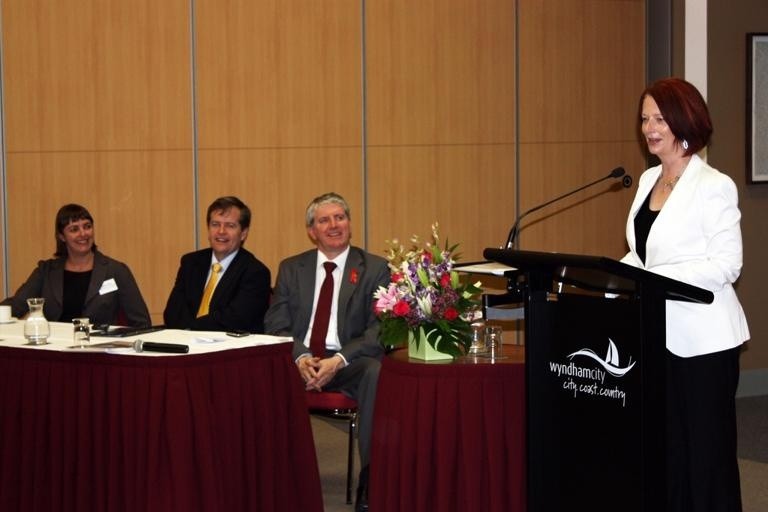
left=370, top=221, right=486, bottom=357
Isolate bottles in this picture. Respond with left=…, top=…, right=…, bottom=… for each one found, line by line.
left=25, top=298, right=51, bottom=346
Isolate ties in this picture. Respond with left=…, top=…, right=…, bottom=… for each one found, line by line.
left=196, top=264, right=222, bottom=319
left=310, top=262, right=337, bottom=360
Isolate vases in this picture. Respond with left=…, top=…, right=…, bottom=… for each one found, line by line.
left=406, top=324, right=456, bottom=362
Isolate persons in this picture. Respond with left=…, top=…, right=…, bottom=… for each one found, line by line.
left=602, top=77, right=751, bottom=512
left=264, top=192, right=390, bottom=511
left=0, top=203, right=152, bottom=331
left=162, top=196, right=271, bottom=334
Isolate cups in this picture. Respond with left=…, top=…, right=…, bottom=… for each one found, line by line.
left=71, top=318, right=92, bottom=345
left=469, top=324, right=504, bottom=357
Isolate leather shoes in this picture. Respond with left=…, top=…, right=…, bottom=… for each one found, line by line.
left=356, top=477, right=369, bottom=512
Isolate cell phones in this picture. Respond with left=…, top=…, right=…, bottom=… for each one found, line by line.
left=226, top=331, right=251, bottom=338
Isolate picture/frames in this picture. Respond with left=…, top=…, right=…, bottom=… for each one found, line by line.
left=743, top=32, right=768, bottom=184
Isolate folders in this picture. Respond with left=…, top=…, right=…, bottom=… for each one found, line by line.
left=88, top=327, right=164, bottom=337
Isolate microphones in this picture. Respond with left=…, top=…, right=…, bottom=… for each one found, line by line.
left=132, top=338, right=190, bottom=354
left=505, top=166, right=626, bottom=250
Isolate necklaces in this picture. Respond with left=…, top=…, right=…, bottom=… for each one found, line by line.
left=660, top=156, right=689, bottom=194
